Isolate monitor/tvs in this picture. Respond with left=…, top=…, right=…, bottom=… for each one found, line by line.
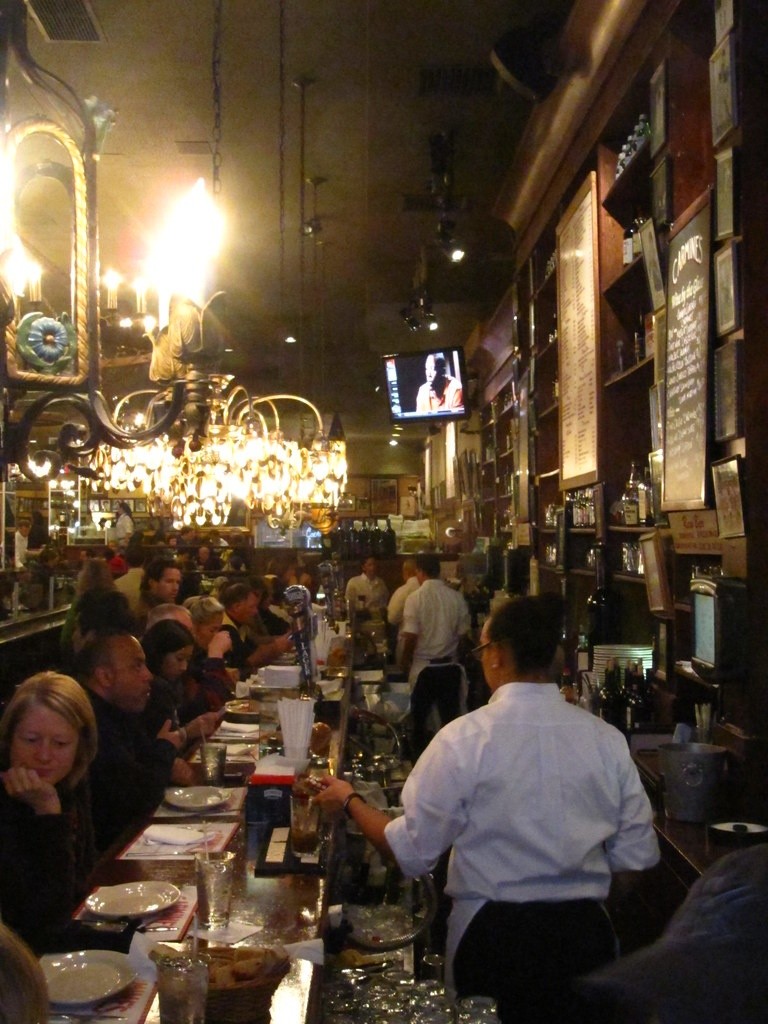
left=380, top=344, right=472, bottom=424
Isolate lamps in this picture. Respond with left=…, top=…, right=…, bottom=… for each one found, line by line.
left=0, top=0, right=350, bottom=537
left=435, top=234, right=465, bottom=264
left=430, top=165, right=452, bottom=204
left=399, top=289, right=440, bottom=333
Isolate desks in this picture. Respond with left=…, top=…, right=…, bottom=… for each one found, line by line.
left=29, top=645, right=350, bottom=1024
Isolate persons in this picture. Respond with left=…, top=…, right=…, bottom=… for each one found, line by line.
left=312, top=597, right=659, bottom=1024
left=0, top=923, right=50, bottom=1024
left=416, top=353, right=464, bottom=412
left=572, top=742, right=768, bottom=1024
left=0, top=671, right=99, bottom=953
left=0, top=502, right=321, bottom=853
left=344, top=555, right=565, bottom=760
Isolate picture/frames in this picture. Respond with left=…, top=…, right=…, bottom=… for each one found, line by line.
left=649, top=156, right=673, bottom=228
left=112, top=499, right=123, bottom=513
left=100, top=498, right=111, bottom=512
left=134, top=498, right=147, bottom=513
left=338, top=494, right=356, bottom=512
left=648, top=58, right=668, bottom=157
left=88, top=498, right=100, bottom=512
left=649, top=452, right=665, bottom=521
left=648, top=383, right=663, bottom=450
left=356, top=497, right=369, bottom=511
left=639, top=530, right=677, bottom=622
left=654, top=306, right=666, bottom=385
left=638, top=217, right=666, bottom=314
left=123, top=499, right=135, bottom=513
left=370, top=477, right=398, bottom=517
left=708, top=0, right=748, bottom=544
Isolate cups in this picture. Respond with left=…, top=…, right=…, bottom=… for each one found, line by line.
left=290, top=794, right=320, bottom=857
left=319, top=970, right=502, bottom=1024
left=200, top=743, right=227, bottom=786
left=194, top=851, right=236, bottom=928
left=156, top=951, right=211, bottom=1024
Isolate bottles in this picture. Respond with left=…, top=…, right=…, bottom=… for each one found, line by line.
left=618, top=462, right=653, bottom=526
left=560, top=669, right=579, bottom=705
left=615, top=114, right=651, bottom=179
left=546, top=504, right=564, bottom=528
left=574, top=631, right=592, bottom=685
left=634, top=300, right=654, bottom=364
left=564, top=487, right=596, bottom=527
left=622, top=200, right=649, bottom=267
left=595, top=657, right=656, bottom=744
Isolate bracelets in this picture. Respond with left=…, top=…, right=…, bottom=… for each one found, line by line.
left=342, top=793, right=366, bottom=819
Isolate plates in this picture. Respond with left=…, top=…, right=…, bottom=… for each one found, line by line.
left=165, top=786, right=232, bottom=810
left=225, top=700, right=258, bottom=714
left=85, top=881, right=182, bottom=916
left=36, top=950, right=139, bottom=1005
left=593, top=645, right=653, bottom=688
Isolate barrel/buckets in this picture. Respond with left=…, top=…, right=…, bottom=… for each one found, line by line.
left=657, top=743, right=728, bottom=823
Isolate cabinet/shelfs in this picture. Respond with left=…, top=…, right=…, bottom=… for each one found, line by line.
left=477, top=380, right=517, bottom=548
left=534, top=525, right=660, bottom=583
left=48, top=436, right=81, bottom=536
left=534, top=269, right=559, bottom=421
left=599, top=134, right=657, bottom=387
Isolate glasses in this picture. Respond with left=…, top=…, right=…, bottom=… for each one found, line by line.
left=471, top=641, right=491, bottom=660
